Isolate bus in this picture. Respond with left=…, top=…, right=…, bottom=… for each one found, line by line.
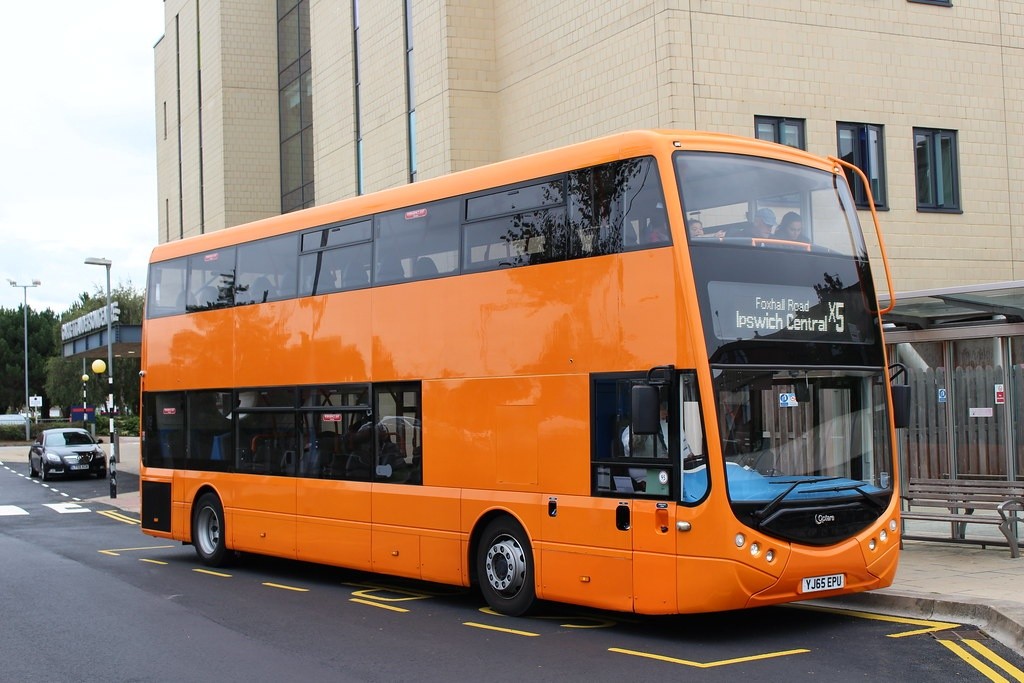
left=136, top=125, right=914, bottom=618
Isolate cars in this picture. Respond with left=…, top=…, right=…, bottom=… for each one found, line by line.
left=0, top=414, right=27, bottom=427
left=28, top=427, right=108, bottom=482
left=17, top=409, right=35, bottom=420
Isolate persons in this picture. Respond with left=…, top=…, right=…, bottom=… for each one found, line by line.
left=646, top=208, right=669, bottom=243
left=622, top=395, right=696, bottom=491
left=346, top=422, right=408, bottom=480
left=775, top=211, right=811, bottom=243
left=688, top=220, right=724, bottom=237
left=726, top=208, right=778, bottom=238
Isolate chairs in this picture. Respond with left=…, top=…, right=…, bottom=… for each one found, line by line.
left=176, top=257, right=439, bottom=315
left=610, top=417, right=631, bottom=458
left=626, top=218, right=639, bottom=246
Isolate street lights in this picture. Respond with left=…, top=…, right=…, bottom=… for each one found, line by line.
left=83, top=258, right=117, bottom=500
left=5, top=278, right=43, bottom=441
left=82, top=372, right=88, bottom=431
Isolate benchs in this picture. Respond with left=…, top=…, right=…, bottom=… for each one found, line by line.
left=899, top=479, right=1024, bottom=558
left=160, top=424, right=401, bottom=476
